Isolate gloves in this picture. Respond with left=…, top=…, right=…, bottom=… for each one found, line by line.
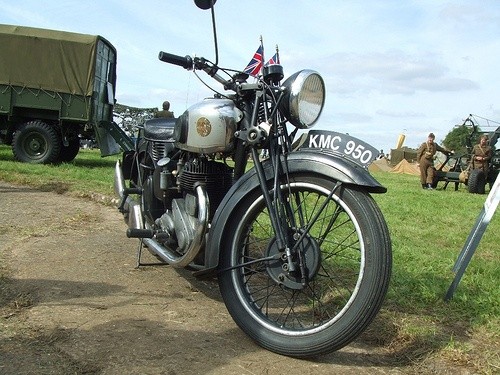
left=451, top=150, right=455, bottom=154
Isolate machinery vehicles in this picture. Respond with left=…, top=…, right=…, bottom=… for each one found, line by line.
left=422, top=112, right=500, bottom=192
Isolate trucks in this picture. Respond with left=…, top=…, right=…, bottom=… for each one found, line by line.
left=0, top=21, right=121, bottom=167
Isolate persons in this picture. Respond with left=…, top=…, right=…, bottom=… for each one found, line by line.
left=153, top=101, right=174, bottom=118
left=416, top=132, right=452, bottom=190
left=472, top=135, right=492, bottom=172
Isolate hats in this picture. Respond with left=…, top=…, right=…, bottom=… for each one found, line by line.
left=481, top=135, right=489, bottom=140
left=428, top=132, right=435, bottom=138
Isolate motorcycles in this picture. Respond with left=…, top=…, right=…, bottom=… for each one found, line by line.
left=112, top=0, right=394, bottom=359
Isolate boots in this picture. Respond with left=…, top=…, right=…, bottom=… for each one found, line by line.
left=427, top=183, right=434, bottom=190
left=422, top=184, right=427, bottom=189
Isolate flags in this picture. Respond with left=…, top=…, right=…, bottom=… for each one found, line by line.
left=242, top=45, right=261, bottom=78
left=265, top=53, right=277, bottom=65
